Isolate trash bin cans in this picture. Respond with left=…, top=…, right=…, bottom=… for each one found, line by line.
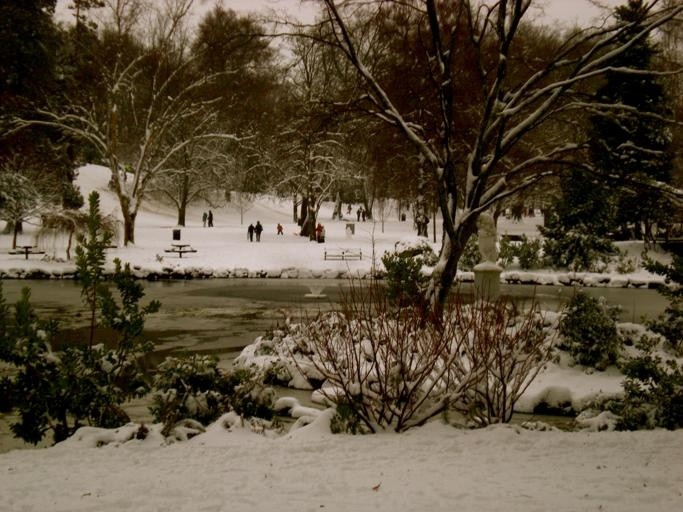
left=401, top=214, right=406, bottom=221
left=346, top=224, right=354, bottom=234
left=173, top=230, right=181, bottom=240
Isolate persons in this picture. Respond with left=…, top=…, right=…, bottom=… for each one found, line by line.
left=315, top=201, right=429, bottom=243
left=203, top=212, right=207, bottom=227
left=207, top=210, right=213, bottom=227
left=248, top=224, right=255, bottom=242
left=254, top=221, right=263, bottom=242
left=277, top=223, right=283, bottom=235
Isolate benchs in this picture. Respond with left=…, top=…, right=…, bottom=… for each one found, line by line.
left=323, top=246, right=363, bottom=261
